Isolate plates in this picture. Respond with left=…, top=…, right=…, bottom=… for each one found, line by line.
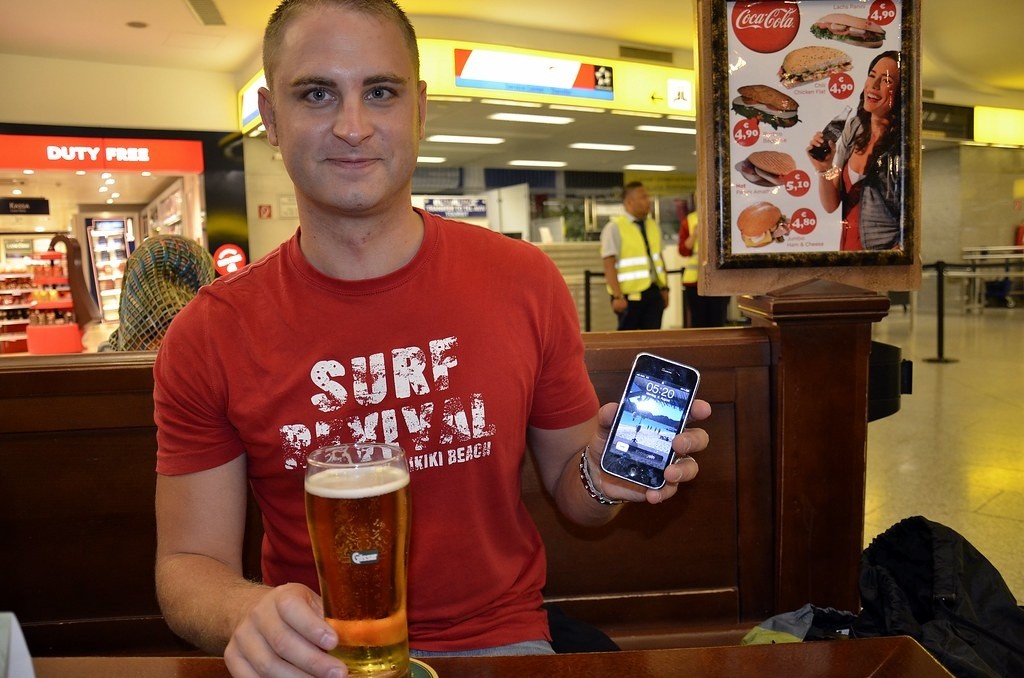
left=409, top=656, right=440, bottom=678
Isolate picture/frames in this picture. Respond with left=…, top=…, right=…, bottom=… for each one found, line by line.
left=709, top=0, right=916, bottom=272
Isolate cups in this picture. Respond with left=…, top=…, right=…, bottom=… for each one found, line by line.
left=303, top=444, right=410, bottom=678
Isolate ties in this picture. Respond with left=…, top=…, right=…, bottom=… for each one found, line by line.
left=633, top=220, right=651, bottom=257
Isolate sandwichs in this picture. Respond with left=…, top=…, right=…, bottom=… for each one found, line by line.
left=777, top=46, right=853, bottom=89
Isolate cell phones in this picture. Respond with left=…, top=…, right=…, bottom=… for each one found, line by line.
left=598, top=352, right=702, bottom=491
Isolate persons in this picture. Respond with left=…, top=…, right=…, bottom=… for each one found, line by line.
left=599, top=180, right=670, bottom=331
left=155, top=0, right=713, bottom=677
left=678, top=188, right=731, bottom=329
left=805, top=50, right=903, bottom=251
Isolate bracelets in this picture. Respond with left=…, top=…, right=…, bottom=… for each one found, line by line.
left=579, top=442, right=632, bottom=505
left=815, top=165, right=842, bottom=181
left=612, top=294, right=624, bottom=299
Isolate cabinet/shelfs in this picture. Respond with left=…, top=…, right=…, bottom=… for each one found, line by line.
left=0, top=252, right=75, bottom=356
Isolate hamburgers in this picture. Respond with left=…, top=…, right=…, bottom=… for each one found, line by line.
left=731, top=85, right=802, bottom=131
left=810, top=14, right=886, bottom=48
left=737, top=201, right=791, bottom=247
left=734, top=151, right=796, bottom=187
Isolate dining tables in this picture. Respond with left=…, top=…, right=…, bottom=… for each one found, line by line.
left=28, top=634, right=955, bottom=678
